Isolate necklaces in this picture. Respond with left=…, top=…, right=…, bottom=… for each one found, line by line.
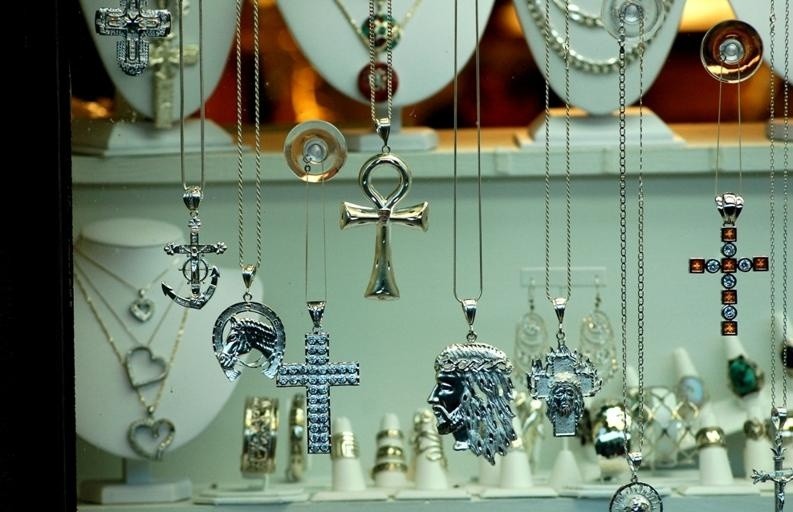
left=71, top=0, right=793, bottom=511
